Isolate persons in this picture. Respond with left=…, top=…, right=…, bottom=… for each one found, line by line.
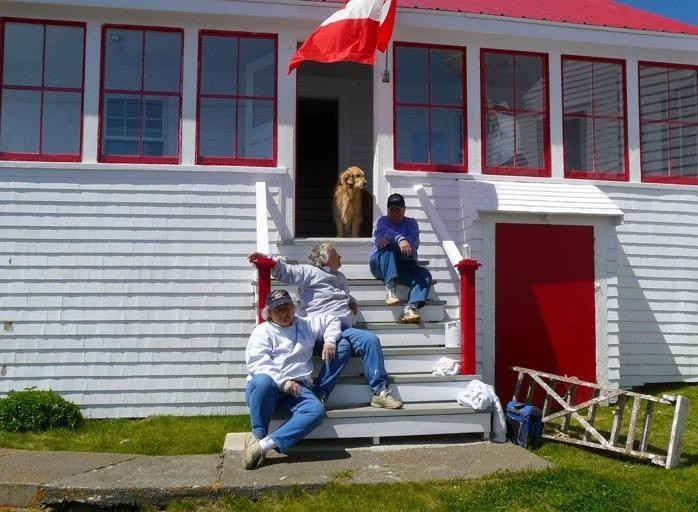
left=246, top=241, right=402, bottom=408
left=369, top=193, right=431, bottom=323
left=242, top=289, right=342, bottom=468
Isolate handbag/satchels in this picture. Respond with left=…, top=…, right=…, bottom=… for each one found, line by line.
left=504, top=399, right=543, bottom=450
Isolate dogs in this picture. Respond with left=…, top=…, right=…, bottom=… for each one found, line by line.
left=331, top=164, right=373, bottom=238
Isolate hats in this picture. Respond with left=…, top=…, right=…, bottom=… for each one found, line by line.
left=387, top=194, right=405, bottom=210
left=265, top=289, right=293, bottom=311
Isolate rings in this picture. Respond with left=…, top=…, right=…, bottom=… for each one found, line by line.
left=292, top=388, right=297, bottom=390
left=325, top=352, right=329, bottom=354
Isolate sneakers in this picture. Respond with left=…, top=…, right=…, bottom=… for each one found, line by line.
left=384, top=281, right=399, bottom=305
left=370, top=390, right=403, bottom=409
left=245, top=438, right=265, bottom=470
left=399, top=302, right=422, bottom=322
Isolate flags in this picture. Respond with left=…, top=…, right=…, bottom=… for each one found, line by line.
left=286, top=0, right=397, bottom=76
